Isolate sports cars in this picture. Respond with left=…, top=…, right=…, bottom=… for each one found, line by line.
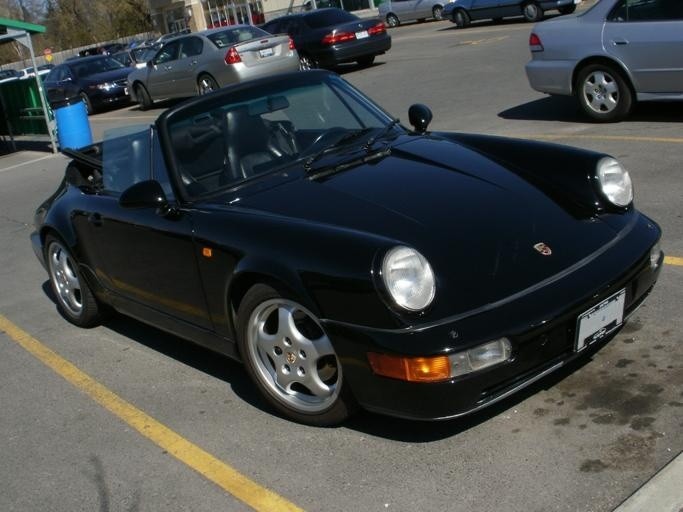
left=30, top=69, right=667, bottom=427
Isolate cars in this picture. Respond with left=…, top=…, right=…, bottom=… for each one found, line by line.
left=438, top=0, right=581, bottom=29
left=523, top=0, right=682, bottom=122
left=126, top=25, right=299, bottom=109
left=376, top=0, right=455, bottom=27
left=258, top=6, right=392, bottom=73
left=0, top=10, right=263, bottom=115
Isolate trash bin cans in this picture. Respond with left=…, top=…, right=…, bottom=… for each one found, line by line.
left=50, top=95, right=93, bottom=154
